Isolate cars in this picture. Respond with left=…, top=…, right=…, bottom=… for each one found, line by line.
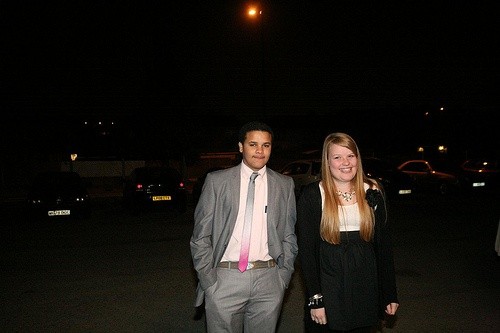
left=459, top=159, right=500, bottom=195
left=123, top=165, right=188, bottom=213
left=397, top=158, right=469, bottom=197
left=25, top=171, right=94, bottom=223
left=275, top=158, right=385, bottom=206
left=192, top=164, right=235, bottom=204
left=360, top=158, right=413, bottom=201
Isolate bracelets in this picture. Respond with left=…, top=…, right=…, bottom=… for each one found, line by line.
left=310, top=293, right=322, bottom=305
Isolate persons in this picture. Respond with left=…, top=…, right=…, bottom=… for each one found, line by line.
left=190, top=120, right=299, bottom=333
left=294, top=133, right=399, bottom=333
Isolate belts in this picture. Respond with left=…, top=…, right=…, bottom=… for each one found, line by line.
left=216, top=259, right=276, bottom=270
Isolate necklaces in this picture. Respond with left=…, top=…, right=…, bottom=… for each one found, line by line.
left=338, top=191, right=356, bottom=202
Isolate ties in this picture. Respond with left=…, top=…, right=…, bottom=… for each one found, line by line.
left=237, top=171, right=261, bottom=273
